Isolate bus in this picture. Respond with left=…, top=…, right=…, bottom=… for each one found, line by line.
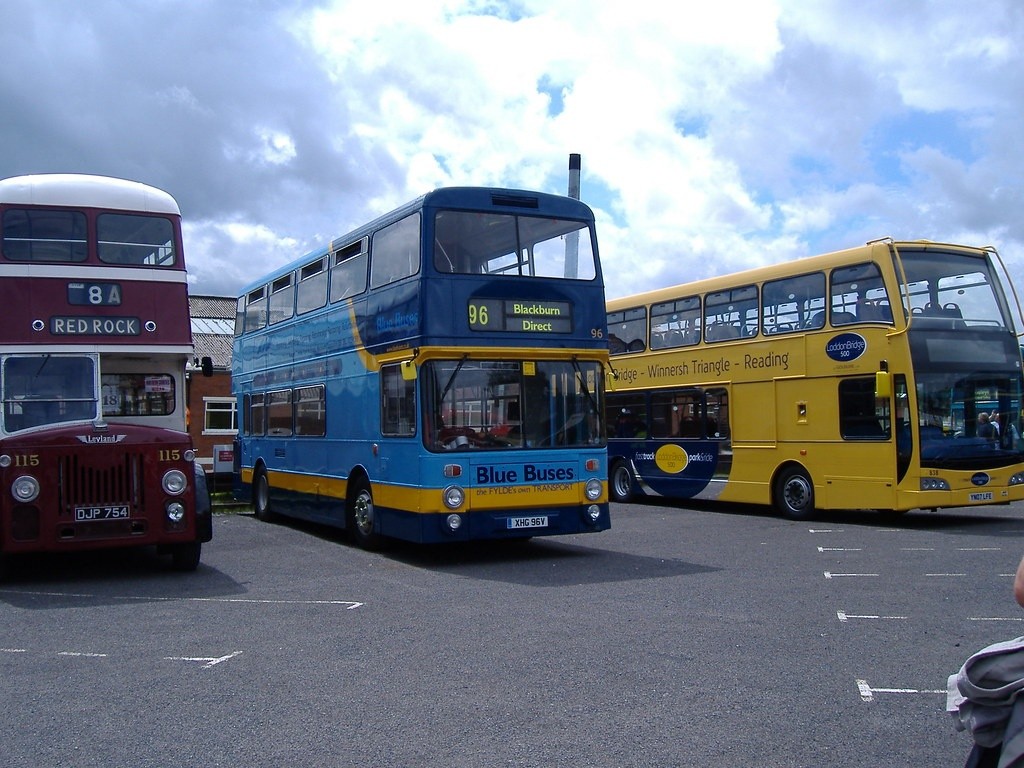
left=604, top=235, right=1024, bottom=521
left=232, top=185, right=612, bottom=548
left=0, top=173, right=213, bottom=576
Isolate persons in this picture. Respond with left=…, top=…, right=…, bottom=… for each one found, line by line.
left=978, top=409, right=1020, bottom=450
left=1014, top=556, right=1024, bottom=609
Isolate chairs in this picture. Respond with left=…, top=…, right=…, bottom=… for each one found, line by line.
left=677, top=415, right=695, bottom=438
left=877, top=297, right=893, bottom=321
left=912, top=308, right=925, bottom=317
left=856, top=297, right=880, bottom=320
left=925, top=302, right=942, bottom=316
left=20, top=394, right=60, bottom=429
left=706, top=311, right=855, bottom=341
left=943, top=303, right=963, bottom=318
left=651, top=329, right=699, bottom=349
